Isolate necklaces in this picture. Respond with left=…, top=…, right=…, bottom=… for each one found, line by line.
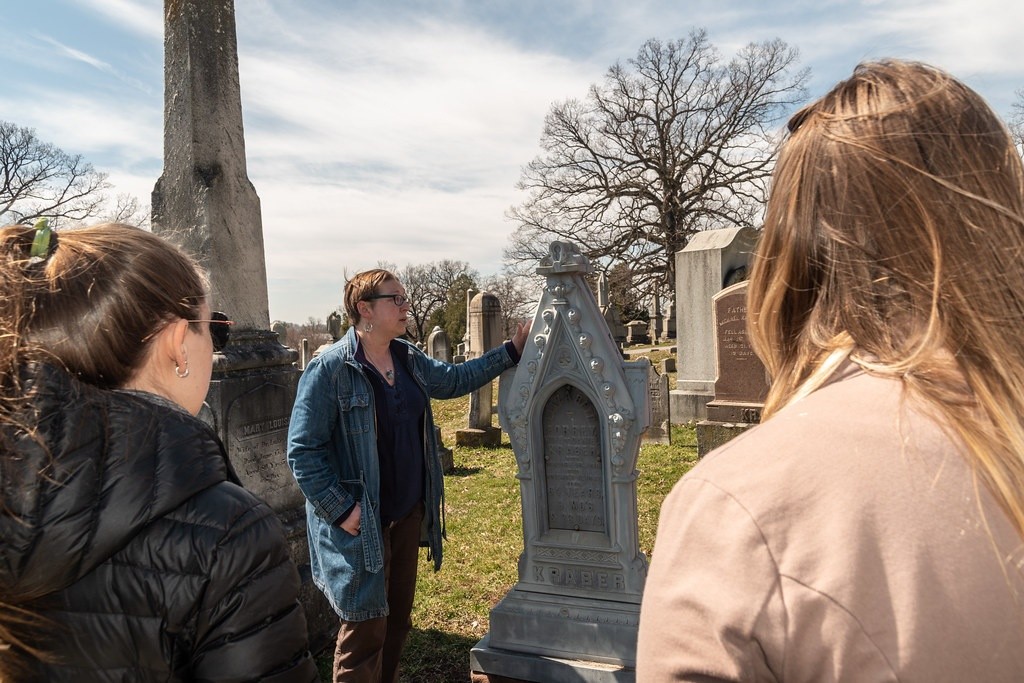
left=381, top=368, right=393, bottom=379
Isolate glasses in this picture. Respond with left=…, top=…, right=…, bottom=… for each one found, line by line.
left=364, top=294, right=412, bottom=306
left=141, top=312, right=237, bottom=344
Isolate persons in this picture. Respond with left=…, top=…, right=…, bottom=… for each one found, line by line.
left=0, top=221, right=321, bottom=683
left=634, top=56, right=1023, bottom=683
left=288, top=269, right=531, bottom=683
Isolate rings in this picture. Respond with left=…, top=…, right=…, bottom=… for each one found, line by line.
left=357, top=525, right=361, bottom=531
